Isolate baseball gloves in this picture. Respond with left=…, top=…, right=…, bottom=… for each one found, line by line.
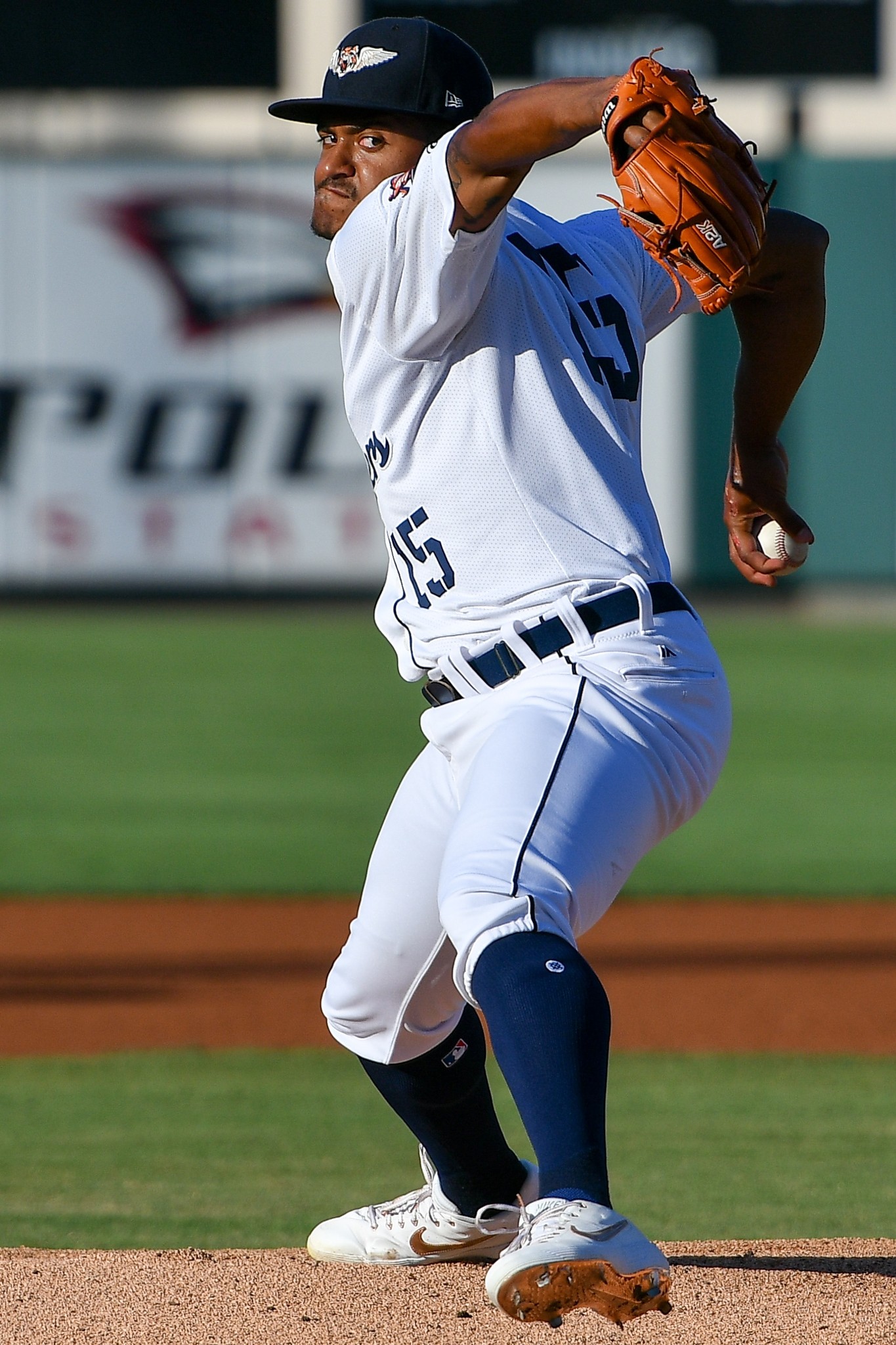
left=596, top=56, right=769, bottom=318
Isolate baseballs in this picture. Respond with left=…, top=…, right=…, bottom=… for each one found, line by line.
left=756, top=520, right=808, bottom=577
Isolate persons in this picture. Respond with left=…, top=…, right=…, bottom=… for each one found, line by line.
left=267, top=17, right=830, bottom=1324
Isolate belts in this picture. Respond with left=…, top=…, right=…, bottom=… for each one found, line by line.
left=421, top=573, right=688, bottom=707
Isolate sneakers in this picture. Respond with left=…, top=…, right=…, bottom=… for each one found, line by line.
left=306, top=1150, right=540, bottom=1266
left=484, top=1195, right=675, bottom=1331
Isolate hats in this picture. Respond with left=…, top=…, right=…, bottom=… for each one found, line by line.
left=269, top=14, right=495, bottom=126
